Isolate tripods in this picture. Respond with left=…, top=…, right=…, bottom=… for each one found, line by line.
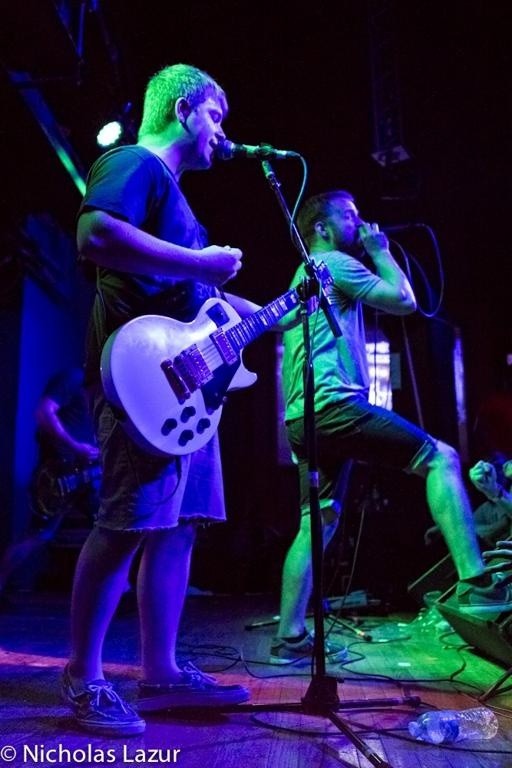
left=155, top=160, right=420, bottom=768
left=245, top=558, right=372, bottom=642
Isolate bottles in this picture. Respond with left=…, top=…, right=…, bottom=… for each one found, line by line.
left=408, top=707, right=498, bottom=744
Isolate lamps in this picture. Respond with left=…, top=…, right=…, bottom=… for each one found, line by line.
left=95, top=116, right=124, bottom=151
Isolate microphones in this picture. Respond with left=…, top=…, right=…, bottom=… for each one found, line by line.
left=358, top=222, right=411, bottom=247
left=214, top=140, right=299, bottom=161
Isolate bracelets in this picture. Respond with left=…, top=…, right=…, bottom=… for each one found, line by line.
left=486, top=484, right=504, bottom=506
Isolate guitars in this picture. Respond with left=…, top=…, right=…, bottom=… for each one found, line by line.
left=100, top=257, right=335, bottom=457
left=31, top=457, right=102, bottom=520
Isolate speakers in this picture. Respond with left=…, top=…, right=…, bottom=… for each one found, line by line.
left=436, top=533, right=512, bottom=668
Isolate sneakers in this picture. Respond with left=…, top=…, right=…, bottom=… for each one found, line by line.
left=61, top=662, right=147, bottom=737
left=456, top=571, right=512, bottom=614
left=133, top=660, right=253, bottom=711
left=267, top=632, right=349, bottom=671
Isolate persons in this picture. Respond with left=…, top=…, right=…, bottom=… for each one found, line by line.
left=20, top=349, right=107, bottom=616
left=266, top=188, right=511, bottom=667
left=468, top=460, right=511, bottom=519
left=53, top=61, right=326, bottom=739
